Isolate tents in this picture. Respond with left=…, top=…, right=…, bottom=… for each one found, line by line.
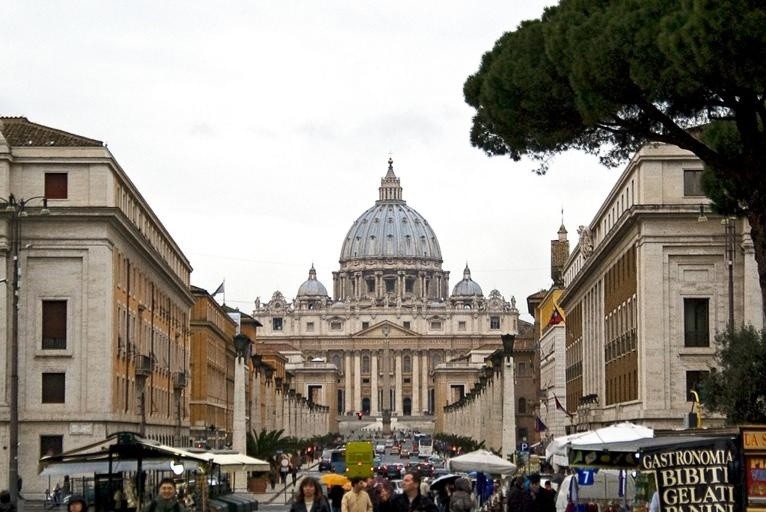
left=545, top=422, right=655, bottom=512
left=445, top=448, right=518, bottom=474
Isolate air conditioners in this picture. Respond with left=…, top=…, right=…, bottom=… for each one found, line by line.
left=135, top=354, right=155, bottom=378
left=173, top=371, right=186, bottom=390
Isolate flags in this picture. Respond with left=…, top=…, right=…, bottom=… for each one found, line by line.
left=548, top=310, right=565, bottom=326
left=535, top=417, right=547, bottom=432
left=210, top=281, right=223, bottom=301
left=548, top=395, right=576, bottom=415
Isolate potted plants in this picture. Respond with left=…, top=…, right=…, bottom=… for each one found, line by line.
left=289, top=432, right=340, bottom=463
left=246, top=427, right=286, bottom=494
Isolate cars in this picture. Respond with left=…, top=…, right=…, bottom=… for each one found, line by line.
left=391, top=480, right=405, bottom=495
left=373, top=432, right=433, bottom=479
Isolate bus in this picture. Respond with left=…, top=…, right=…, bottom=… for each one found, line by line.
left=319, top=448, right=346, bottom=475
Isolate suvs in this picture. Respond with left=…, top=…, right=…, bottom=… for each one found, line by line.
left=319, top=462, right=330, bottom=473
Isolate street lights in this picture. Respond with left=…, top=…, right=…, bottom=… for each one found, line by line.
left=0, top=192, right=52, bottom=510
left=439, top=333, right=518, bottom=462
left=698, top=202, right=734, bottom=335
left=228, top=332, right=328, bottom=495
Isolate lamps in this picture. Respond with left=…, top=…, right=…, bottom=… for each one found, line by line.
left=232, top=332, right=329, bottom=412
left=443, top=332, right=515, bottom=413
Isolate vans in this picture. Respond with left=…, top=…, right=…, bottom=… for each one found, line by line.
left=345, top=441, right=375, bottom=479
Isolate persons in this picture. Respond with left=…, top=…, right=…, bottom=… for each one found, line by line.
left=565, top=493, right=661, bottom=512
left=67, top=495, right=89, bottom=512
left=433, top=440, right=459, bottom=459
left=53, top=484, right=62, bottom=503
left=269, top=465, right=298, bottom=489
left=144, top=478, right=190, bottom=512
left=290, top=470, right=557, bottom=512
